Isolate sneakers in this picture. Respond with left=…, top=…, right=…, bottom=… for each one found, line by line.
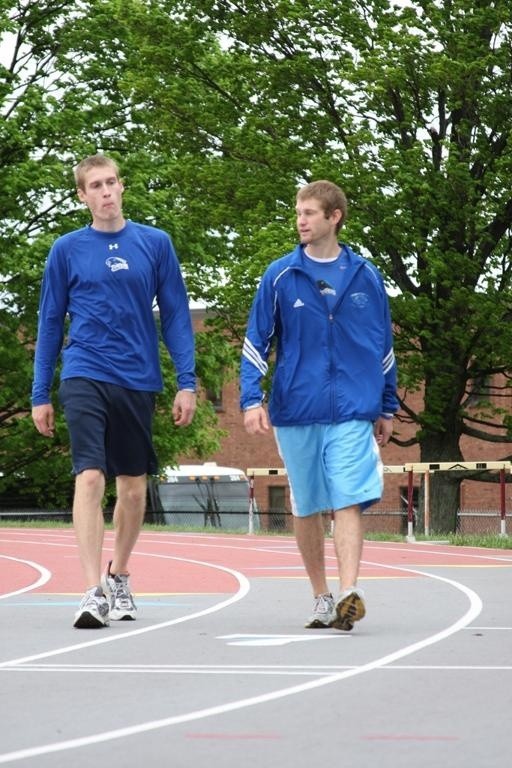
left=73, top=585, right=111, bottom=626
left=304, top=592, right=338, bottom=628
left=327, top=589, right=366, bottom=630
left=101, top=559, right=138, bottom=620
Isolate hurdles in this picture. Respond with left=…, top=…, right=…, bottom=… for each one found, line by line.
left=246, top=460, right=512, bottom=544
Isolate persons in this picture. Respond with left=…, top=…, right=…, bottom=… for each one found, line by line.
left=237, top=178, right=402, bottom=633
left=29, top=153, right=199, bottom=631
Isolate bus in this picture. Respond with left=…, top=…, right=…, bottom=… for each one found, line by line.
left=156, top=458, right=263, bottom=534
